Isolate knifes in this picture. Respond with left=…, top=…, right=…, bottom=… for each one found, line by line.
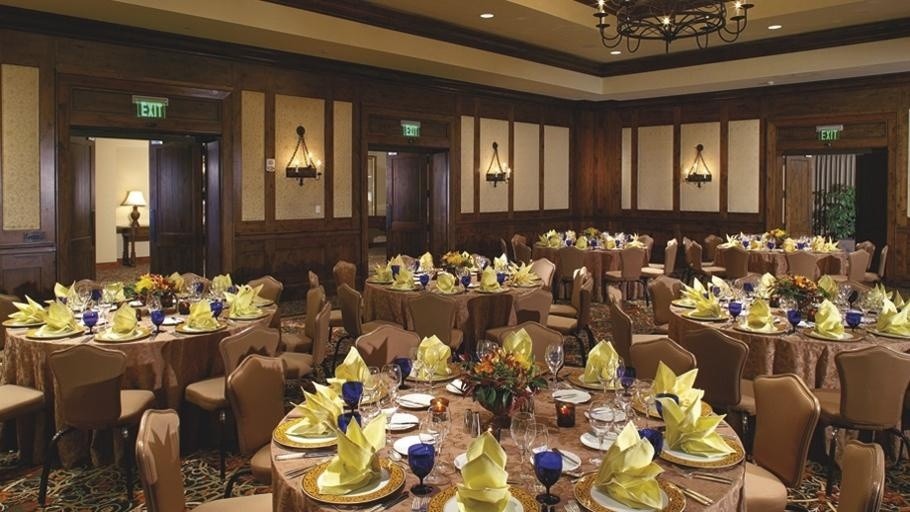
left=400, top=398, right=424, bottom=405
left=668, top=481, right=715, bottom=506
left=286, top=460, right=318, bottom=479
left=276, top=451, right=334, bottom=461
left=364, top=490, right=409, bottom=512
left=392, top=422, right=417, bottom=425
left=552, top=447, right=578, bottom=464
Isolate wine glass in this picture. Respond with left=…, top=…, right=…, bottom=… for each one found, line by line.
left=361, top=366, right=380, bottom=418
left=89, top=288, right=103, bottom=309
left=615, top=239, right=621, bottom=248
left=83, top=311, right=99, bottom=335
left=179, top=276, right=209, bottom=304
left=565, top=240, right=572, bottom=246
left=338, top=412, right=363, bottom=433
left=743, top=282, right=754, bottom=297
left=476, top=339, right=491, bottom=363
left=151, top=310, right=168, bottom=333
left=420, top=275, right=430, bottom=293
left=524, top=423, right=549, bottom=481
left=482, top=342, right=498, bottom=356
left=408, top=443, right=434, bottom=497
left=637, top=429, right=665, bottom=461
left=728, top=302, right=742, bottom=324
left=341, top=380, right=363, bottom=411
left=655, top=393, right=679, bottom=422
left=145, top=294, right=160, bottom=314
left=591, top=239, right=598, bottom=250
left=797, top=241, right=805, bottom=250
left=377, top=387, right=401, bottom=448
left=380, top=364, right=402, bottom=411
left=637, top=378, right=657, bottom=429
left=106, top=279, right=120, bottom=309
left=409, top=346, right=423, bottom=394
left=462, top=276, right=470, bottom=293
left=534, top=450, right=563, bottom=506
left=743, top=240, right=749, bottom=250
left=588, top=400, right=615, bottom=467
left=596, top=362, right=615, bottom=406
left=767, top=241, right=775, bottom=253
left=846, top=309, right=861, bottom=337
left=545, top=343, right=565, bottom=402
left=392, top=356, right=411, bottom=390
left=787, top=309, right=801, bottom=335
left=79, top=286, right=93, bottom=313
left=359, top=392, right=380, bottom=424
left=420, top=416, right=450, bottom=485
left=426, top=405, right=457, bottom=476
left=617, top=367, right=636, bottom=397
left=421, top=347, right=441, bottom=397
left=832, top=281, right=885, bottom=328
left=614, top=377, right=637, bottom=427
left=510, top=411, right=536, bottom=469
left=210, top=301, right=223, bottom=321
left=497, top=271, right=506, bottom=287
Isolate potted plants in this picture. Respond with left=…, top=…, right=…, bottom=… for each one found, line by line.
left=812, top=186, right=856, bottom=252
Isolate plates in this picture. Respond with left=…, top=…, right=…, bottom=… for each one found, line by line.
left=272, top=417, right=338, bottom=449
left=25, top=324, right=84, bottom=338
left=551, top=389, right=591, bottom=406
left=732, top=313, right=791, bottom=335
left=682, top=307, right=730, bottom=320
left=431, top=286, right=463, bottom=295
left=407, top=363, right=466, bottom=381
left=428, top=480, right=540, bottom=512
left=630, top=389, right=712, bottom=419
left=2, top=318, right=48, bottom=328
left=528, top=447, right=582, bottom=473
left=567, top=370, right=628, bottom=391
left=300, top=457, right=406, bottom=504
left=473, top=285, right=510, bottom=294
left=393, top=435, right=425, bottom=456
left=94, top=322, right=150, bottom=342
left=175, top=319, right=227, bottom=333
left=229, top=306, right=270, bottom=320
left=446, top=378, right=469, bottom=396
left=580, top=430, right=621, bottom=452
left=454, top=451, right=470, bottom=471
left=256, top=294, right=274, bottom=308
left=509, top=280, right=541, bottom=287
left=583, top=407, right=626, bottom=424
left=161, top=316, right=184, bottom=325
left=383, top=413, right=418, bottom=432
left=671, top=297, right=698, bottom=308
left=397, top=393, right=437, bottom=409
left=367, top=278, right=395, bottom=285
left=803, top=326, right=865, bottom=342
left=657, top=430, right=745, bottom=469
left=385, top=283, right=422, bottom=292
left=315, top=382, right=388, bottom=407
left=865, top=325, right=910, bottom=341
left=573, top=470, right=687, bottom=512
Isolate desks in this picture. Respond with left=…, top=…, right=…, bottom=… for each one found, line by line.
left=117, top=225, right=151, bottom=268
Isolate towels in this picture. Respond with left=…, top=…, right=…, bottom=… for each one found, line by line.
left=709, top=276, right=733, bottom=299
left=182, top=297, right=215, bottom=329
left=817, top=274, right=839, bottom=300
left=878, top=290, right=905, bottom=315
left=442, top=249, right=461, bottom=269
left=757, top=272, right=777, bottom=300
left=575, top=235, right=589, bottom=248
left=814, top=299, right=844, bottom=341
left=868, top=282, right=893, bottom=310
left=564, top=230, right=576, bottom=241
left=746, top=296, right=777, bottom=334
left=453, top=431, right=510, bottom=510
left=168, top=271, right=186, bottom=290
left=493, top=253, right=508, bottom=271
left=7, top=294, right=41, bottom=325
left=875, top=297, right=909, bottom=336
left=413, top=335, right=451, bottom=377
left=462, top=250, right=476, bottom=268
left=813, top=235, right=830, bottom=254
left=374, top=263, right=391, bottom=281
left=626, top=232, right=640, bottom=247
left=236, top=281, right=267, bottom=304
left=53, top=281, right=81, bottom=301
left=799, top=235, right=810, bottom=243
left=386, top=254, right=405, bottom=271
left=107, top=302, right=142, bottom=339
left=689, top=287, right=722, bottom=319
left=103, top=280, right=124, bottom=301
left=325, top=346, right=370, bottom=387
left=827, top=236, right=843, bottom=251
left=285, top=382, right=344, bottom=438
left=481, top=265, right=500, bottom=293
left=538, top=229, right=557, bottom=248
left=678, top=276, right=706, bottom=305
left=228, top=284, right=263, bottom=318
left=593, top=419, right=667, bottom=510
left=211, top=273, right=232, bottom=294
left=435, top=270, right=456, bottom=294
left=579, top=339, right=620, bottom=388
left=645, top=359, right=705, bottom=414
left=507, top=265, right=533, bottom=286
left=502, top=328, right=536, bottom=361
left=602, top=233, right=616, bottom=249
left=783, top=238, right=798, bottom=253
left=391, top=264, right=414, bottom=289
left=508, top=261, right=535, bottom=272
left=37, top=298, right=77, bottom=331
left=657, top=392, right=739, bottom=458
left=317, top=414, right=387, bottom=494
left=741, top=231, right=758, bottom=250
left=417, top=252, right=433, bottom=272
left=725, top=233, right=739, bottom=246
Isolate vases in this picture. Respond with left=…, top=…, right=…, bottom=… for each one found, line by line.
left=146, top=291, right=164, bottom=317
left=447, top=268, right=459, bottom=286
left=774, top=239, right=784, bottom=250
left=480, top=403, right=515, bottom=441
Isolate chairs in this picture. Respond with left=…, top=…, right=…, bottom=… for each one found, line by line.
left=278, top=299, right=333, bottom=394
left=550, top=265, right=590, bottom=319
left=548, top=271, right=595, bottom=369
left=641, top=240, right=677, bottom=300
left=406, top=292, right=465, bottom=364
left=354, top=323, right=422, bottom=372
left=0, top=382, right=48, bottom=466
left=64, top=278, right=101, bottom=296
left=683, top=326, right=758, bottom=455
left=484, top=287, right=554, bottom=345
left=499, top=237, right=508, bottom=257
left=723, top=245, right=749, bottom=282
left=863, top=243, right=889, bottom=289
left=783, top=249, right=819, bottom=281
left=529, top=257, right=557, bottom=292
left=628, top=337, right=697, bottom=386
left=685, top=240, right=727, bottom=289
left=637, top=234, right=654, bottom=262
left=558, top=245, right=585, bottom=301
left=0, top=292, right=21, bottom=349
left=331, top=282, right=404, bottom=379
left=533, top=247, right=561, bottom=302
left=134, top=408, right=274, bottom=511
left=647, top=237, right=679, bottom=270
left=224, top=353, right=288, bottom=501
left=182, top=324, right=282, bottom=483
left=37, top=343, right=157, bottom=507
left=703, top=234, right=725, bottom=262
left=498, top=320, right=566, bottom=369
left=471, top=252, right=492, bottom=266
left=856, top=240, right=876, bottom=272
left=828, top=248, right=871, bottom=290
left=328, top=259, right=357, bottom=344
left=180, top=271, right=213, bottom=296
left=277, top=268, right=327, bottom=352
left=514, top=239, right=532, bottom=269
left=806, top=342, right=910, bottom=498
left=739, top=372, right=822, bottom=510
left=681, top=235, right=716, bottom=284
left=602, top=246, right=650, bottom=308
left=608, top=300, right=670, bottom=371
left=511, top=232, right=527, bottom=261
left=833, top=435, right=886, bottom=512
left=246, top=275, right=284, bottom=328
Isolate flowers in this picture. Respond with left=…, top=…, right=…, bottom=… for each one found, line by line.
left=769, top=228, right=791, bottom=240
left=460, top=346, right=550, bottom=413
left=766, top=274, right=820, bottom=315
left=582, top=227, right=603, bottom=240
left=440, top=251, right=475, bottom=271
left=124, top=272, right=185, bottom=296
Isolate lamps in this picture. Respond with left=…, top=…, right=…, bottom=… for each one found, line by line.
left=684, top=142, right=713, bottom=189
left=285, top=124, right=322, bottom=187
left=120, top=189, right=147, bottom=229
left=485, top=141, right=513, bottom=189
left=574, top=0, right=755, bottom=63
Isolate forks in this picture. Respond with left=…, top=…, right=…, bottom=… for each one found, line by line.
left=565, top=470, right=600, bottom=477
left=670, top=465, right=733, bottom=486
